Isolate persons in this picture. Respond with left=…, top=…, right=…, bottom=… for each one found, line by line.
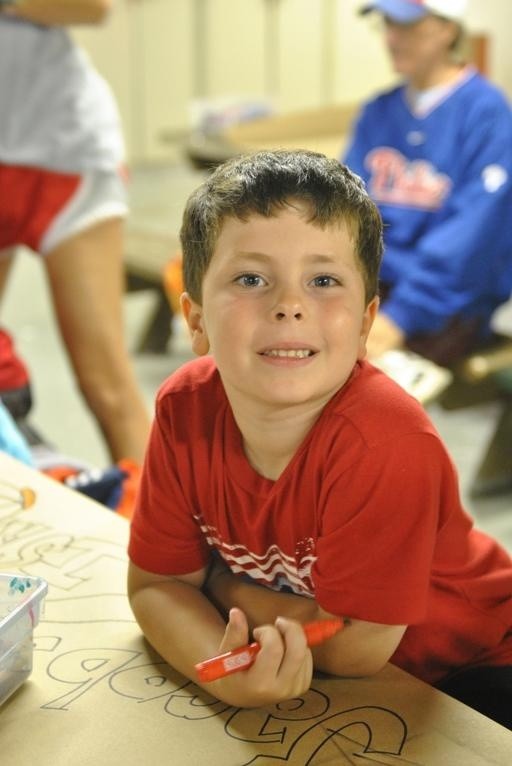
left=333, top=0, right=512, bottom=360
left=126, top=148, right=512, bottom=729
left=1, top=0, right=154, bottom=470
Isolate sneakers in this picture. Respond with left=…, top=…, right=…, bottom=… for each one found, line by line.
left=107, top=456, right=149, bottom=522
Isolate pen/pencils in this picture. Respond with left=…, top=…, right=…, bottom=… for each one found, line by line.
left=193, top=616, right=351, bottom=683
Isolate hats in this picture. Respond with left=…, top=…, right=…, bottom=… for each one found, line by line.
left=357, top=0, right=471, bottom=32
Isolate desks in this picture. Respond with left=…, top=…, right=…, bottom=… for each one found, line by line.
left=0, top=442, right=510, bottom=765
